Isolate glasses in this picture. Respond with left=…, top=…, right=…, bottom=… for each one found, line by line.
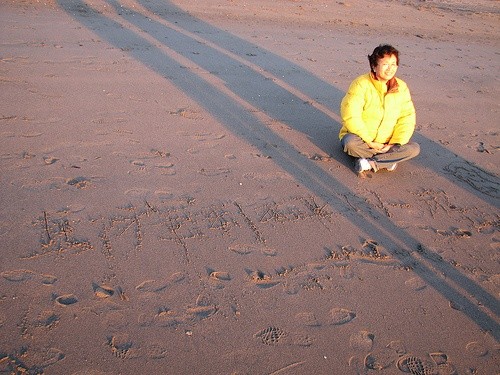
left=378, top=63, right=398, bottom=70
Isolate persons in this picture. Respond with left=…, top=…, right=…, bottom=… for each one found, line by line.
left=338, top=42, right=421, bottom=174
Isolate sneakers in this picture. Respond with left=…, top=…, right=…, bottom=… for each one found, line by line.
left=387, top=163, right=399, bottom=171
left=355, top=158, right=373, bottom=174
